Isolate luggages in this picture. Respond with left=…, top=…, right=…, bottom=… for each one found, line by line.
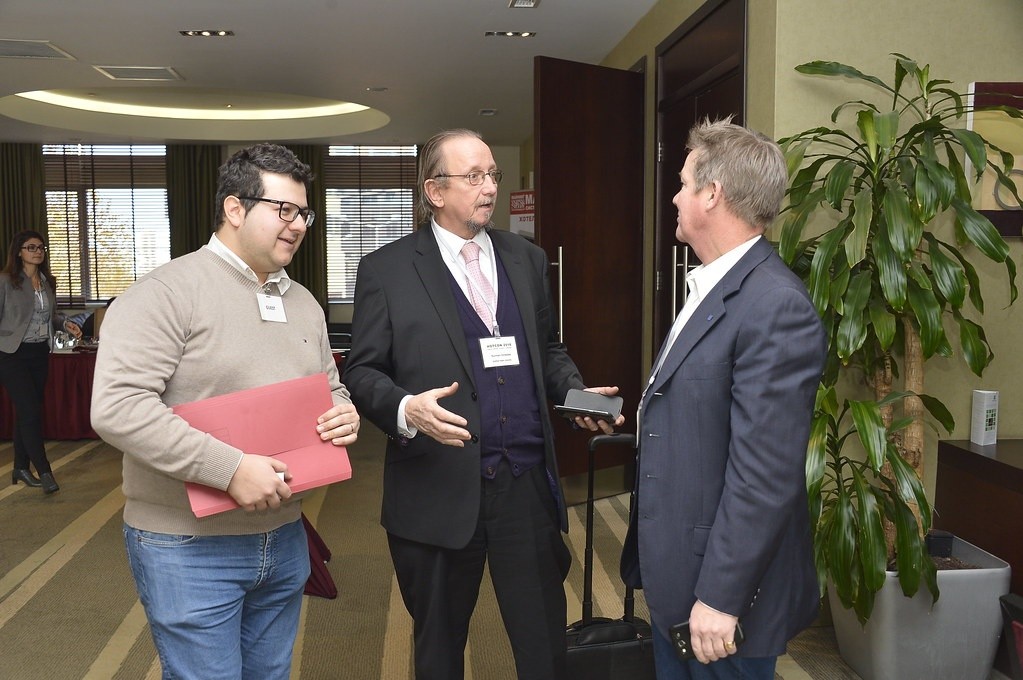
left=565, top=432, right=655, bottom=680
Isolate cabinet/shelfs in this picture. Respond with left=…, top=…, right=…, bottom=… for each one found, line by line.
left=932, top=438, right=1023, bottom=598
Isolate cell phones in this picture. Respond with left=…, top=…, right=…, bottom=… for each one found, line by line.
left=552, top=388, right=623, bottom=425
left=669, top=620, right=745, bottom=662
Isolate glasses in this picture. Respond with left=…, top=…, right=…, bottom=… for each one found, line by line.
left=432, top=169, right=505, bottom=186
left=20, top=244, right=48, bottom=252
left=237, top=196, right=315, bottom=228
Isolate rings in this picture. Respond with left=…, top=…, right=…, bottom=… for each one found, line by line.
left=724, top=640, right=736, bottom=651
left=346, top=423, right=354, bottom=432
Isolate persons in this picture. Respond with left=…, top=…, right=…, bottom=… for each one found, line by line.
left=615, top=114, right=824, bottom=680
left=81, top=297, right=117, bottom=338
left=90, top=143, right=361, bottom=680
left=341, top=128, right=624, bottom=680
left=1, top=231, right=83, bottom=493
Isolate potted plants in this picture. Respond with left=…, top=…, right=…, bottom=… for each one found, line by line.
left=776, top=49, right=1015, bottom=680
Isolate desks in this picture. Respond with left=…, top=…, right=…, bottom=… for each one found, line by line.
left=0, top=347, right=100, bottom=441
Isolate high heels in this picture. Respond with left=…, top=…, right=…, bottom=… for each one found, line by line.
left=38, top=472, right=59, bottom=493
left=11, top=468, right=43, bottom=487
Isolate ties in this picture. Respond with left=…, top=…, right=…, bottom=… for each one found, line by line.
left=460, top=243, right=497, bottom=337
